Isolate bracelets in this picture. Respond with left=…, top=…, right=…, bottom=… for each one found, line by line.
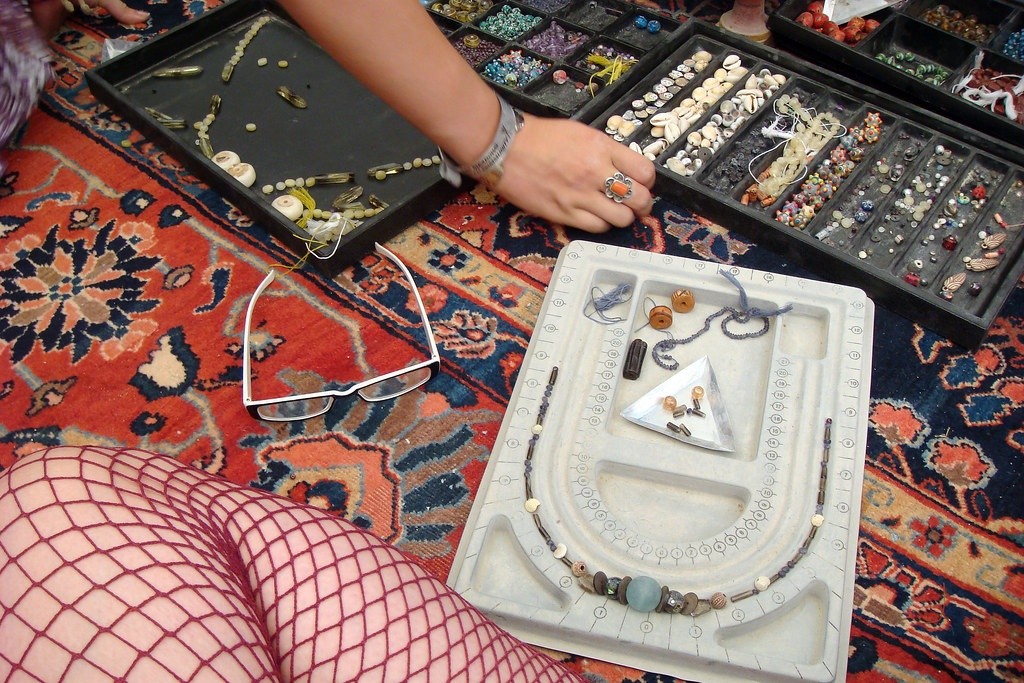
left=438, top=93, right=524, bottom=187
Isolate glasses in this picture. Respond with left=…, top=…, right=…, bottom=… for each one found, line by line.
left=241, top=240, right=441, bottom=423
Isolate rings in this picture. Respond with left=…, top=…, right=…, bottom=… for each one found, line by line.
left=605, top=172, right=632, bottom=203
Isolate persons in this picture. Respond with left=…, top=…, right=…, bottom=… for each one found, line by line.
left=0, top=0, right=655, bottom=233
left=0, top=445, right=579, bottom=683
